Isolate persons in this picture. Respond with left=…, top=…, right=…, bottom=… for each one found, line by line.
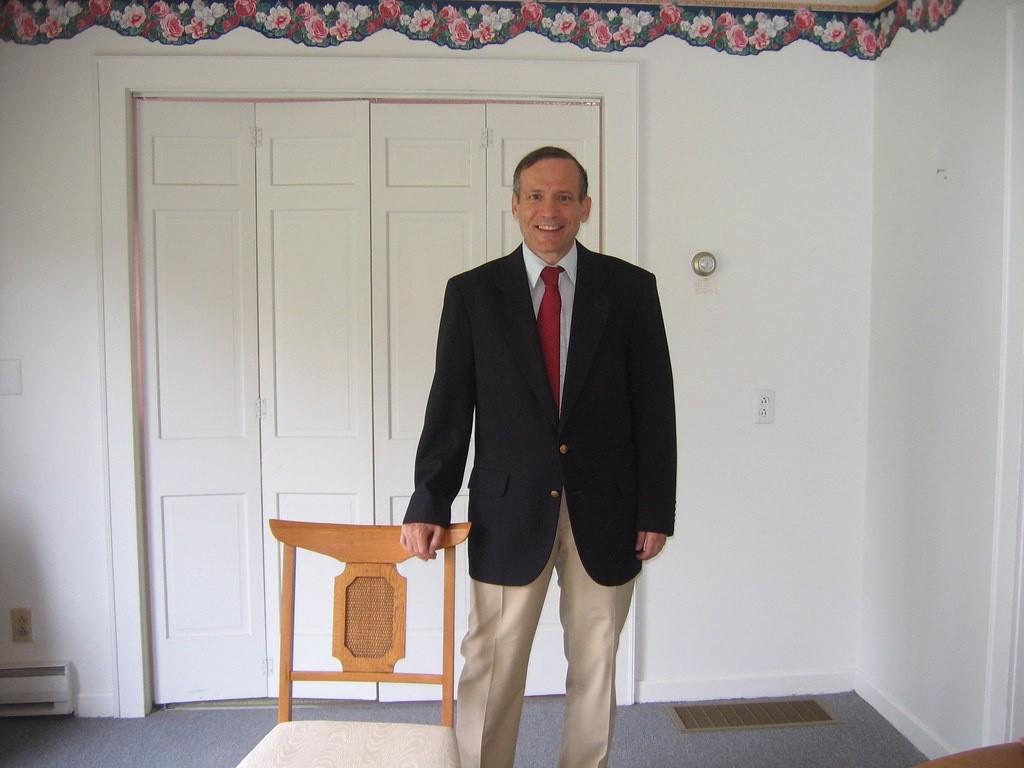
left=400, top=147, right=678, bottom=768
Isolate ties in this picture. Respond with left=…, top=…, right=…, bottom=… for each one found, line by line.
left=538, top=266, right=565, bottom=414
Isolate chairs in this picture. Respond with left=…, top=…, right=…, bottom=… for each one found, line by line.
left=235, top=518, right=472, bottom=768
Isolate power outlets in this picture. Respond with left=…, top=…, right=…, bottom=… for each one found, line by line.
left=10, top=608, right=32, bottom=643
